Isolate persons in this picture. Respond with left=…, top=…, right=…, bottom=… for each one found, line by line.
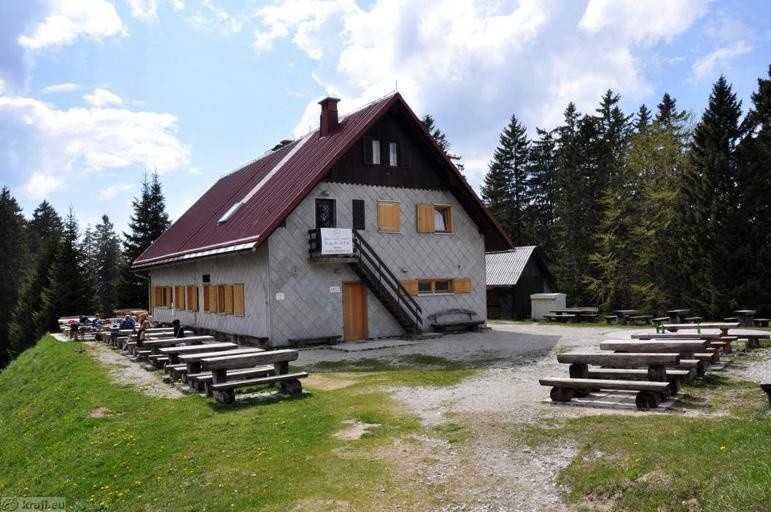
left=67, top=311, right=150, bottom=356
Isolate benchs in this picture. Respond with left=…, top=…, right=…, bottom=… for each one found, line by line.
left=59, top=315, right=310, bottom=405
left=533, top=307, right=771, bottom=411
left=287, top=334, right=342, bottom=348
left=427, top=309, right=488, bottom=335
